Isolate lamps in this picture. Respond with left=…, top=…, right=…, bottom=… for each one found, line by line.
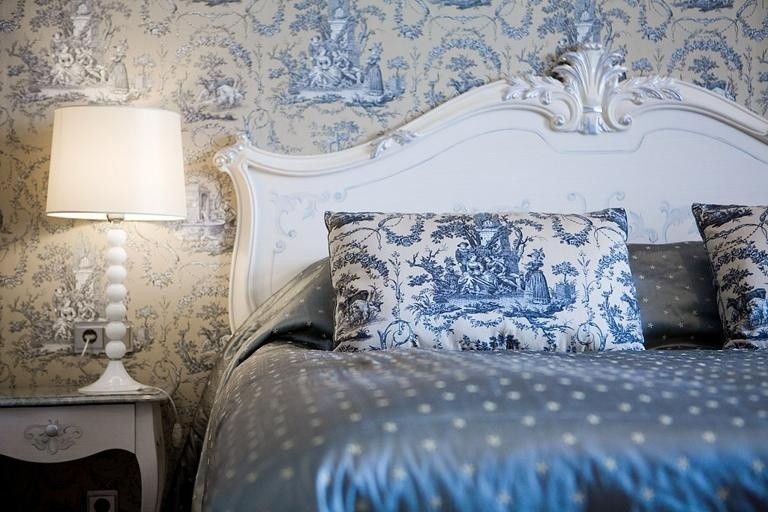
left=43, top=104, right=190, bottom=397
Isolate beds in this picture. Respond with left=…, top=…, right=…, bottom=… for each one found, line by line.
left=211, top=44, right=768, bottom=512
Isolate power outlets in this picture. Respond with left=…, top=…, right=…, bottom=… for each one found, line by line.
left=71, top=320, right=135, bottom=357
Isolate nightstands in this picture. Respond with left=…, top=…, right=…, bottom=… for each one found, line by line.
left=1, top=382, right=172, bottom=512
